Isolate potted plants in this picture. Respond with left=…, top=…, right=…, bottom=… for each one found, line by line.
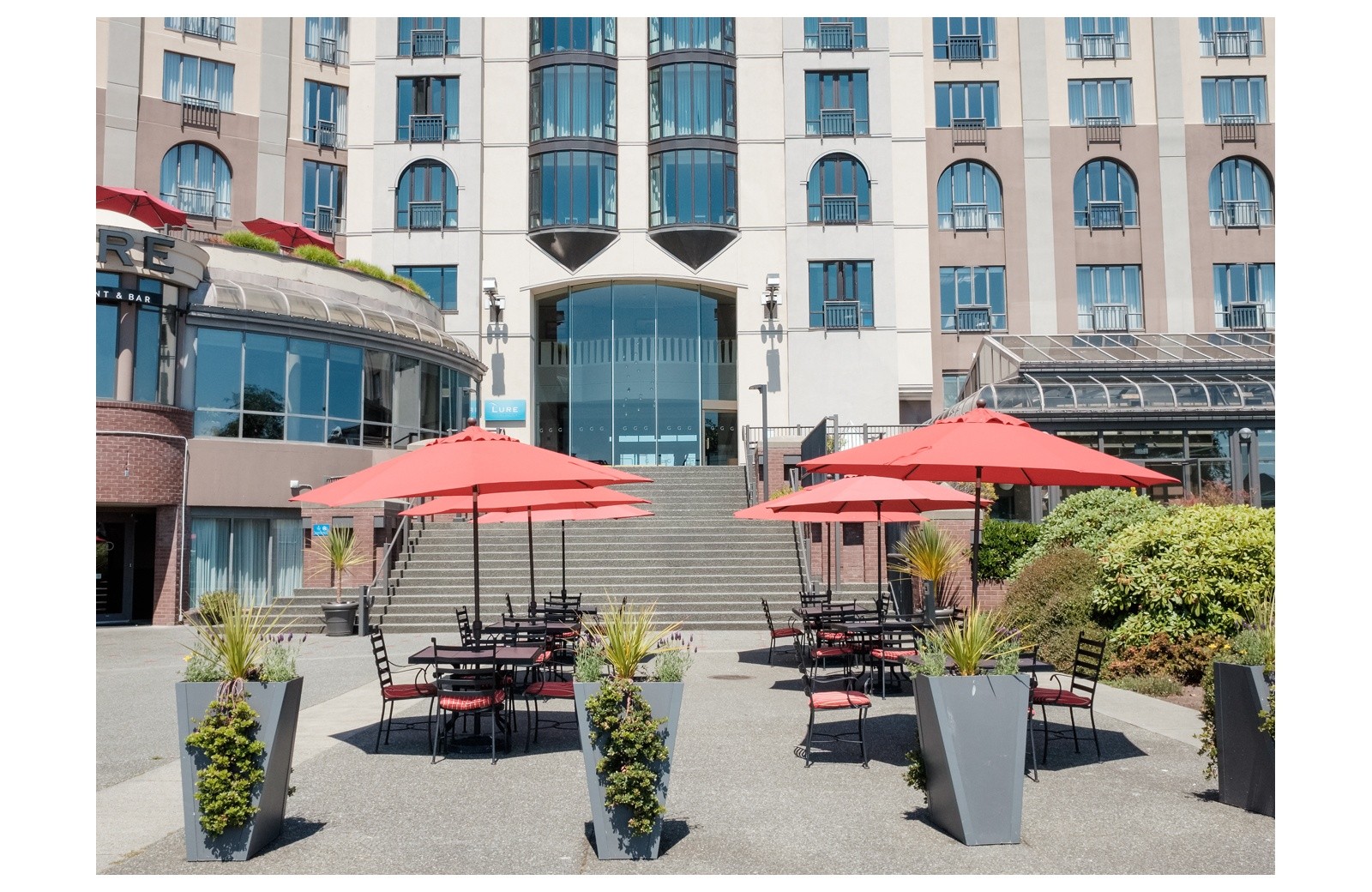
left=169, top=587, right=308, bottom=861
left=897, top=593, right=1040, bottom=846
left=556, top=584, right=699, bottom=860
left=1193, top=586, right=1275, bottom=818
left=196, top=589, right=240, bottom=624
left=301, top=524, right=380, bottom=637
left=884, top=515, right=974, bottom=625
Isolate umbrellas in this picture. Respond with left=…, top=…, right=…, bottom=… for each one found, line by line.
left=733, top=475, right=995, bottom=685
left=97, top=185, right=194, bottom=230
left=241, top=218, right=344, bottom=260
left=796, top=399, right=1181, bottom=612
left=396, top=487, right=652, bottom=682
left=287, top=417, right=654, bottom=737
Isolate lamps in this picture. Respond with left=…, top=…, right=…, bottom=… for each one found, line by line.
left=1239, top=428, right=1252, bottom=439
left=760, top=273, right=782, bottom=322
left=482, top=277, right=506, bottom=321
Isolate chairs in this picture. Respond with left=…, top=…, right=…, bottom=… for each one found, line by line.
left=367, top=591, right=627, bottom=765
left=760, top=588, right=1106, bottom=766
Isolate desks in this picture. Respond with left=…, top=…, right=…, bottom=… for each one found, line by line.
left=831, top=622, right=924, bottom=692
left=408, top=646, right=542, bottom=754
left=485, top=622, right=581, bottom=682
left=905, top=655, right=1056, bottom=676
left=536, top=607, right=596, bottom=649
left=792, top=605, right=869, bottom=659
